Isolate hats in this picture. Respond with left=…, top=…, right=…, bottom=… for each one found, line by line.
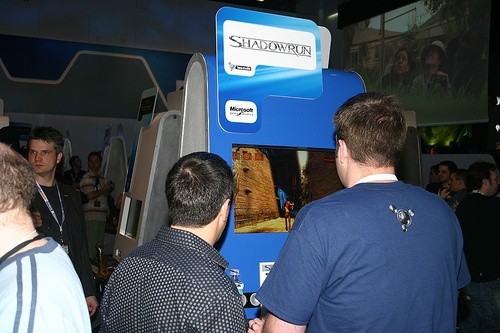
left=422, top=40, right=449, bottom=59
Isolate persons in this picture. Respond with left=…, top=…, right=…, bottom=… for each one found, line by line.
left=284, top=197, right=293, bottom=231
left=376, top=41, right=457, bottom=98
left=0, top=125, right=113, bottom=333
left=425, top=161, right=500, bottom=333
left=249, top=93, right=471, bottom=333
left=99, top=151, right=249, bottom=333
